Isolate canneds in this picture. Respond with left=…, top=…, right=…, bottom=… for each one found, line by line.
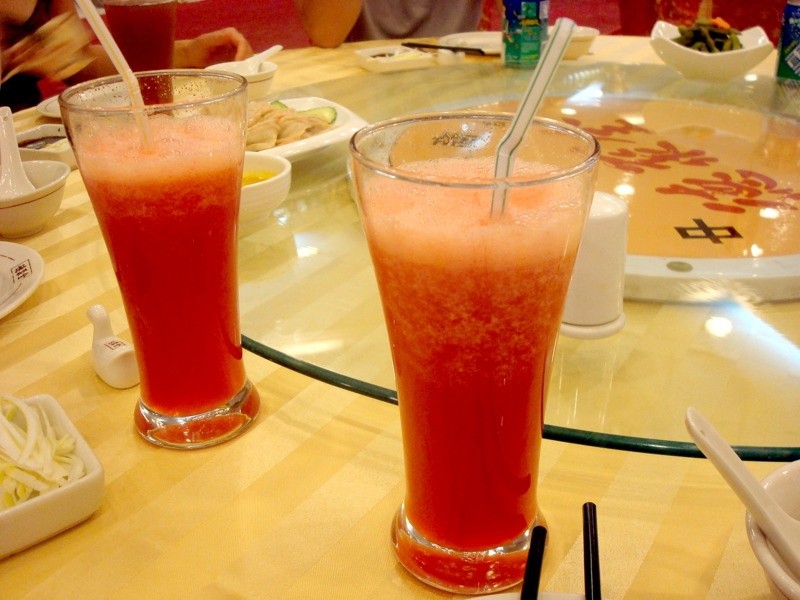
left=776, top=1, right=800, bottom=89
left=501, top=0, right=549, bottom=69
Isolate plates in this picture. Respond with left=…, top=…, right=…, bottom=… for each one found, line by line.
left=38, top=94, right=62, bottom=117
left=650, top=19, right=774, bottom=75
left=0, top=241, right=44, bottom=317
left=439, top=31, right=503, bottom=54
left=255, top=96, right=369, bottom=162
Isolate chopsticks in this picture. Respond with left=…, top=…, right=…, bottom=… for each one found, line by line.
left=402, top=42, right=485, bottom=54
left=520, top=502, right=601, bottom=600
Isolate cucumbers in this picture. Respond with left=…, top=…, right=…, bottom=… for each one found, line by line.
left=270, top=99, right=337, bottom=124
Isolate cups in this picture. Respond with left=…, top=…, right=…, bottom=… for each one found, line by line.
left=350, top=113, right=600, bottom=594
left=58, top=69, right=260, bottom=449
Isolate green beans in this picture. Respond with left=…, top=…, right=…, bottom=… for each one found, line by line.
left=671, top=20, right=744, bottom=53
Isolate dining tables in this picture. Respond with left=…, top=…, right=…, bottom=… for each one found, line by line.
left=0, top=26, right=800, bottom=600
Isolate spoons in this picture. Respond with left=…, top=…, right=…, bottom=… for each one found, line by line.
left=0, top=106, right=36, bottom=200
left=229, top=45, right=283, bottom=75
left=685, top=406, right=800, bottom=582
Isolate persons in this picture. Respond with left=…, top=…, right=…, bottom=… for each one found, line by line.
left=300, top=0, right=484, bottom=50
left=1, top=1, right=253, bottom=114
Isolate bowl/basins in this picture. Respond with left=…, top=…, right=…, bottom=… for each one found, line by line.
left=745, top=460, right=800, bottom=600
left=16, top=124, right=76, bottom=169
left=548, top=25, right=600, bottom=59
left=204, top=61, right=276, bottom=98
left=354, top=45, right=432, bottom=73
left=239, top=151, right=292, bottom=216
left=0, top=394, right=105, bottom=560
left=0, top=161, right=71, bottom=238
